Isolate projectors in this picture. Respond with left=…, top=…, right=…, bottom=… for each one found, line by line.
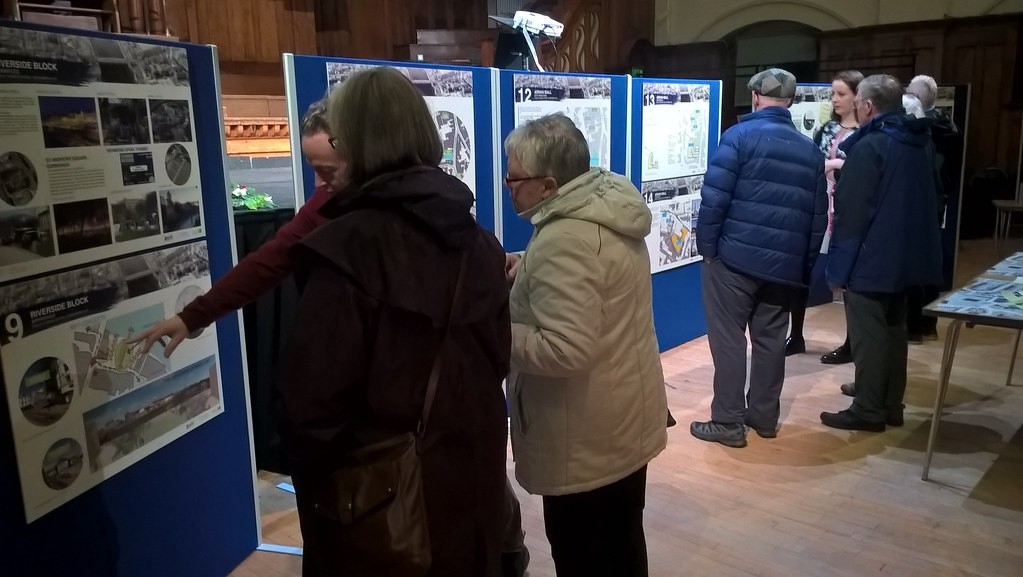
left=513, top=10, right=564, bottom=37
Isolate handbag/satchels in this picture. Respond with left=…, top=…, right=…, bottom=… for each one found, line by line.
left=320, top=431, right=441, bottom=577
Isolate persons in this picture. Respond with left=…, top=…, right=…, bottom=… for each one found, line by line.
left=896, top=72, right=963, bottom=343
left=274, top=66, right=530, bottom=577
left=784, top=68, right=865, bottom=363
left=503, top=108, right=676, bottom=576
left=123, top=96, right=367, bottom=357
left=820, top=73, right=944, bottom=432
left=689, top=65, right=830, bottom=448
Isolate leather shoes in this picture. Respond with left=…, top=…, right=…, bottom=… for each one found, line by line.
left=781, top=334, right=806, bottom=357
left=819, top=344, right=854, bottom=365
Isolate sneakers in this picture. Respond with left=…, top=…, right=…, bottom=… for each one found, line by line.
left=743, top=405, right=777, bottom=439
left=876, top=402, right=905, bottom=427
left=821, top=409, right=886, bottom=435
left=690, top=418, right=747, bottom=450
left=840, top=378, right=855, bottom=394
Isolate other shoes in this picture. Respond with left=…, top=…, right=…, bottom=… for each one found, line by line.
left=908, top=329, right=925, bottom=346
left=923, top=327, right=939, bottom=341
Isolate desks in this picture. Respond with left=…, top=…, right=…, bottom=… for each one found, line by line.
left=921, top=250, right=1023, bottom=482
left=992, top=199, right=1023, bottom=249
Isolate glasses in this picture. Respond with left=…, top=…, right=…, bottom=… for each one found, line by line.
left=903, top=88, right=920, bottom=100
left=505, top=166, right=553, bottom=188
left=327, top=133, right=341, bottom=149
left=852, top=96, right=872, bottom=109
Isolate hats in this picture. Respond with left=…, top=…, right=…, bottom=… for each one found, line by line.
left=746, top=67, right=796, bottom=100
